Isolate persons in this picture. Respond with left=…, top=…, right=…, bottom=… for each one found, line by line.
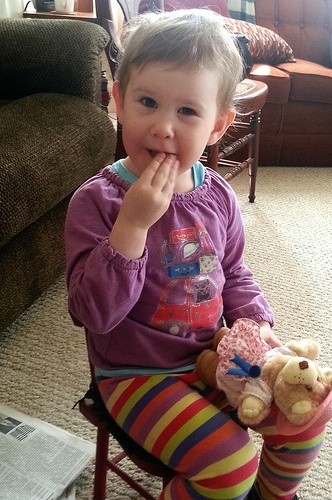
left=65, top=9, right=327, bottom=500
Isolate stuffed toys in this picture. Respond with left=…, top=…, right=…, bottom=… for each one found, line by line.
left=197, top=317, right=332, bottom=436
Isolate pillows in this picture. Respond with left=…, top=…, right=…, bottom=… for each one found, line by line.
left=138, top=0, right=257, bottom=24
left=222, top=17, right=297, bottom=64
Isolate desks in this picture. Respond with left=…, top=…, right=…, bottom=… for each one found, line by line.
left=198, top=79, right=269, bottom=203
left=24, top=0, right=128, bottom=161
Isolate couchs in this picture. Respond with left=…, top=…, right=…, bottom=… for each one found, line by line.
left=203, top=0, right=332, bottom=167
left=0, top=19, right=118, bottom=331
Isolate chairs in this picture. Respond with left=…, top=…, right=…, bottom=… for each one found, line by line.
left=68, top=307, right=248, bottom=500
